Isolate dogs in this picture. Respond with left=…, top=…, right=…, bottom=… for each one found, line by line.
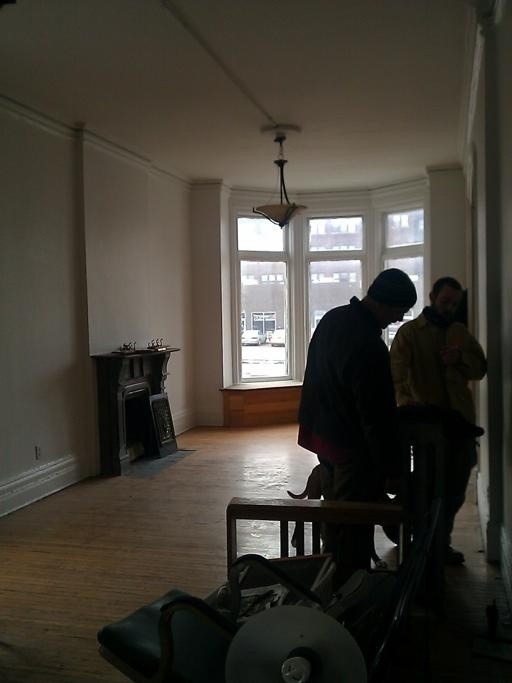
left=287, top=462, right=432, bottom=571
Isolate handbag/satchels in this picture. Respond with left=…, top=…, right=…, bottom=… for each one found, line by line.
left=96, top=587, right=237, bottom=682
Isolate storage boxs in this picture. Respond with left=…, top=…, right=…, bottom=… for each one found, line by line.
left=426, top=583, right=466, bottom=612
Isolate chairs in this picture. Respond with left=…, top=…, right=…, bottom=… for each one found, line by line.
left=97, top=498, right=441, bottom=683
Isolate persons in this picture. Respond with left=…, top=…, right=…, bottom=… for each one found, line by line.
left=389, top=272, right=487, bottom=566
left=295, top=262, right=421, bottom=566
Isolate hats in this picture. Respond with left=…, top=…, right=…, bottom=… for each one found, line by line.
left=367, top=268, right=417, bottom=308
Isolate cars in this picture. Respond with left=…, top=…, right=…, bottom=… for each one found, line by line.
left=270, top=329, right=285, bottom=346
left=242, top=330, right=267, bottom=345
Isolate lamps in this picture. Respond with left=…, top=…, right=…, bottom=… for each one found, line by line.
left=252, top=124, right=307, bottom=230
left=224, top=596, right=367, bottom=683
